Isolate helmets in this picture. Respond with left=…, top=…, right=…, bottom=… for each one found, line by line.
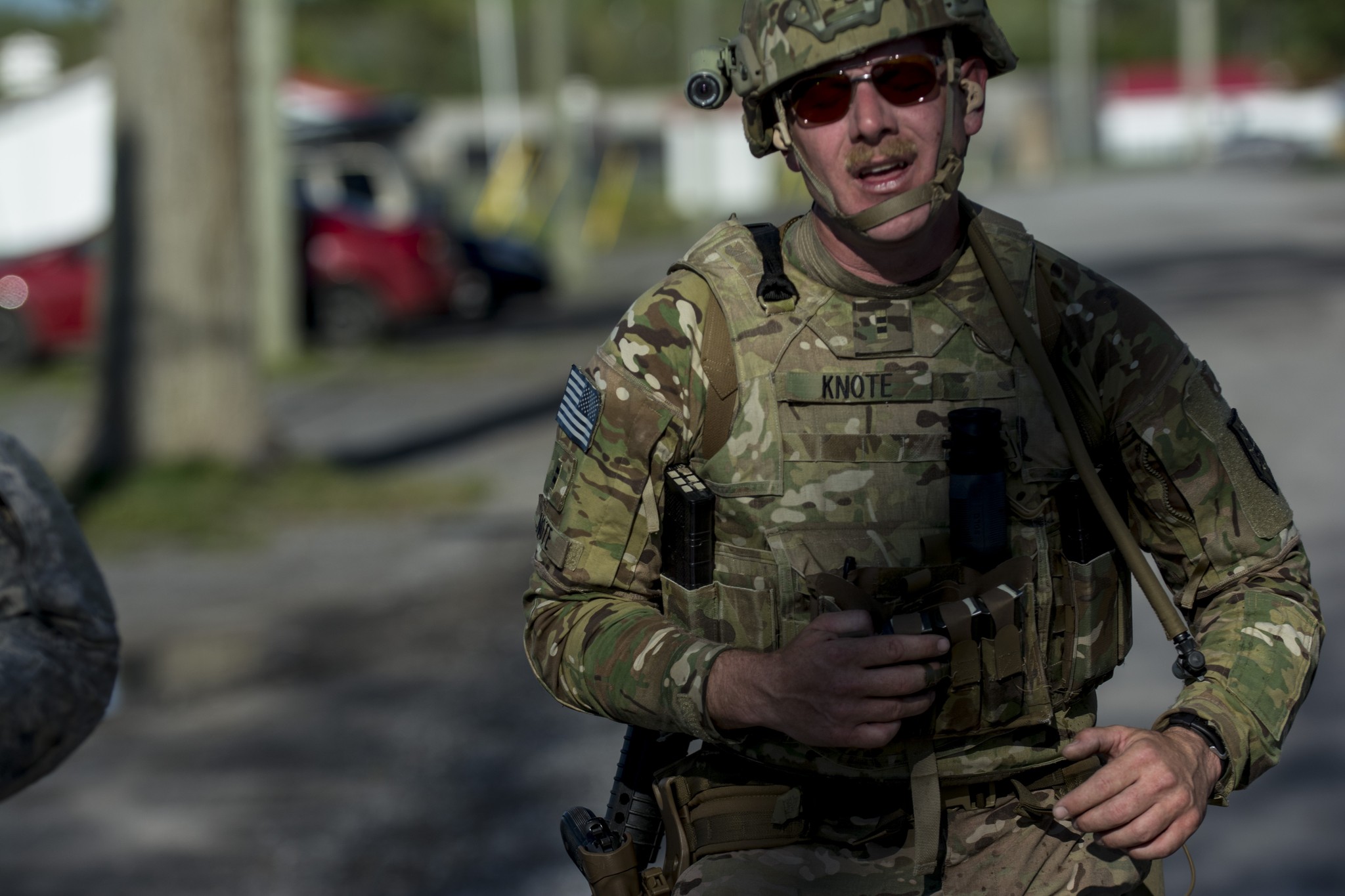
left=731, top=1, right=1019, bottom=158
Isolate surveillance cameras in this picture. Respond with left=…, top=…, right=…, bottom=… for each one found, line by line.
left=685, top=68, right=733, bottom=111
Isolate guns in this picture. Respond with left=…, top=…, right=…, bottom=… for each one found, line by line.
left=600, top=722, right=665, bottom=870
left=558, top=803, right=623, bottom=883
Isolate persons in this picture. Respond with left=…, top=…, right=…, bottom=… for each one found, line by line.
left=523, top=0, right=1324, bottom=896
left=3, top=429, right=124, bottom=813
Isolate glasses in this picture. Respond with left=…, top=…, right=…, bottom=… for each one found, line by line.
left=781, top=54, right=946, bottom=129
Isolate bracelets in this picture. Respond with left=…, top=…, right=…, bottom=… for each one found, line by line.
left=1164, top=707, right=1238, bottom=802
